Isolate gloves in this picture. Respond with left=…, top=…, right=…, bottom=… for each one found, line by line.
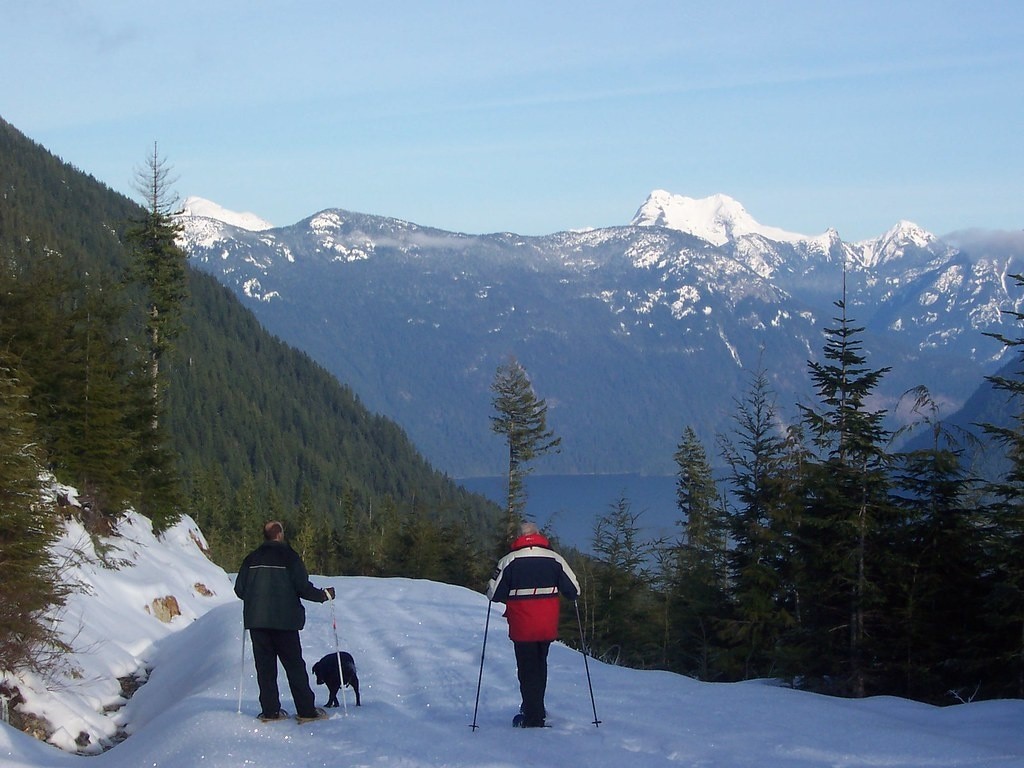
left=323, top=587, right=335, bottom=602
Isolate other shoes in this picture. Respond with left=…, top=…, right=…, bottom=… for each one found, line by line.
left=512, top=713, right=545, bottom=727
left=266, top=711, right=279, bottom=719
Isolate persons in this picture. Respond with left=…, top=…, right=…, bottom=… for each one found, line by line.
left=486, top=522, right=581, bottom=728
left=233, top=521, right=335, bottom=719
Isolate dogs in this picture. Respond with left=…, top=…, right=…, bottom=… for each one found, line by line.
left=312, top=651, right=361, bottom=709
